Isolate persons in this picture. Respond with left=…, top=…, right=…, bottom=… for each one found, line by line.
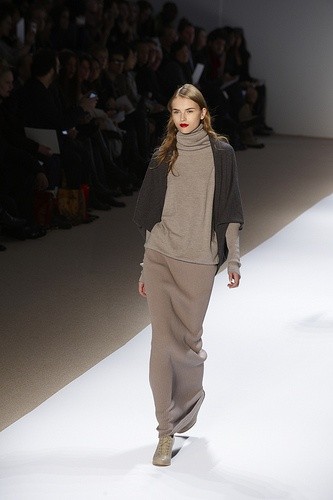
left=134, top=83, right=245, bottom=465
left=0, top=1, right=271, bottom=252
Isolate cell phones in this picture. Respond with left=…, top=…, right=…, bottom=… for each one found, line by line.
left=88, top=92, right=97, bottom=99
left=62, top=130, right=68, bottom=135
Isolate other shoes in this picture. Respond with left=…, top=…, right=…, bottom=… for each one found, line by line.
left=224, top=115, right=272, bottom=151
left=152, top=433, right=175, bottom=465
left=1, top=166, right=142, bottom=252
